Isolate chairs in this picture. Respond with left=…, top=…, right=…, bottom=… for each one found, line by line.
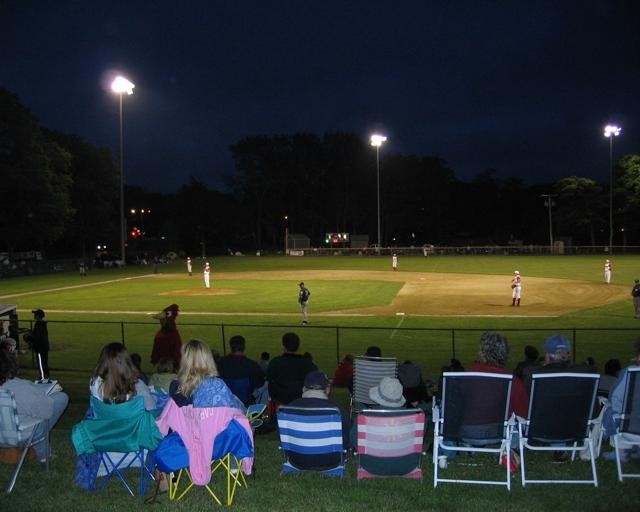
left=431, top=372, right=517, bottom=490
left=599, top=366, right=639, bottom=483
left=353, top=408, right=425, bottom=482
left=70, top=396, right=153, bottom=502
left=153, top=399, right=248, bottom=507
left=515, top=372, right=601, bottom=489
left=276, top=405, right=349, bottom=480
left=0, top=388, right=50, bottom=494
left=347, top=355, right=399, bottom=419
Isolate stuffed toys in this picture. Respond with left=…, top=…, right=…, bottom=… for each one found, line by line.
left=150, top=306, right=183, bottom=374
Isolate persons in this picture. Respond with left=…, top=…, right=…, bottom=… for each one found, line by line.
left=71, top=333, right=352, bottom=492
left=298, top=282, right=309, bottom=325
left=78, top=258, right=85, bottom=276
left=154, top=256, right=160, bottom=273
left=23, top=309, right=49, bottom=379
left=186, top=257, right=193, bottom=277
left=631, top=280, right=640, bottom=319
left=509, top=271, right=521, bottom=307
left=392, top=253, right=398, bottom=271
left=203, top=263, right=211, bottom=288
left=1, top=351, right=69, bottom=468
left=605, top=259, right=611, bottom=284
left=338, top=331, right=640, bottom=476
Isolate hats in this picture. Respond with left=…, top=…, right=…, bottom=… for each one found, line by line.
left=545, top=334, right=569, bottom=354
left=370, top=377, right=406, bottom=408
left=304, top=372, right=328, bottom=389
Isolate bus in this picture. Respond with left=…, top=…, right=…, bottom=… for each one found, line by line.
left=95, top=242, right=110, bottom=258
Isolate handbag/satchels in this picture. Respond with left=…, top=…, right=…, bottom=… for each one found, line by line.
left=154, top=465, right=169, bottom=493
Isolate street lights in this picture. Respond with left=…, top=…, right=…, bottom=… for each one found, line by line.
left=369, top=132, right=389, bottom=254
left=282, top=215, right=289, bottom=257
left=130, top=207, right=151, bottom=264
left=108, top=76, right=138, bottom=267
left=542, top=191, right=560, bottom=254
left=604, top=124, right=624, bottom=255
